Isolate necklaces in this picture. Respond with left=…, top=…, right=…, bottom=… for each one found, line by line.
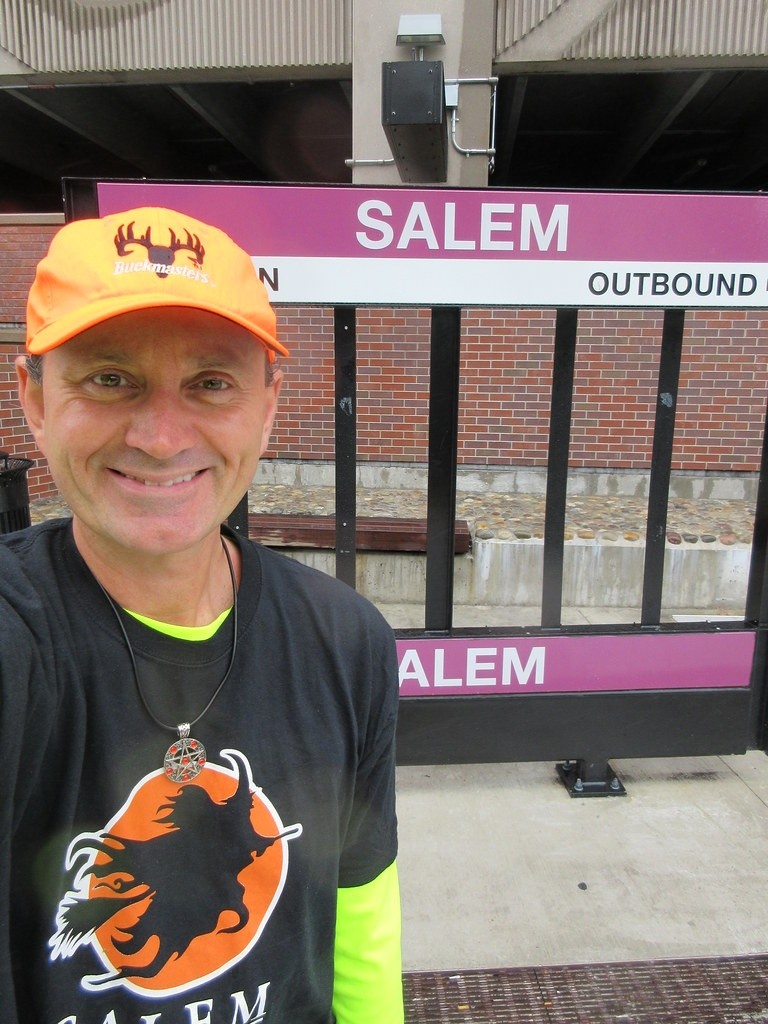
left=95, top=536, right=238, bottom=784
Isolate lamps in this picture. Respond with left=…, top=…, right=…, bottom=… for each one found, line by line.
left=394, top=13, right=447, bottom=61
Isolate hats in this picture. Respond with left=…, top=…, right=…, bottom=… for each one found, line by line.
left=26, top=207, right=289, bottom=365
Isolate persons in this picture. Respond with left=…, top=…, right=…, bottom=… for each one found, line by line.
left=0, top=207, right=404, bottom=1024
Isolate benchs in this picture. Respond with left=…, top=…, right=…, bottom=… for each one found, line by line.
left=224, top=509, right=473, bottom=557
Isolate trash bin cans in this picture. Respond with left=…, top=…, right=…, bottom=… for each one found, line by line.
left=0, top=450, right=34, bottom=534
